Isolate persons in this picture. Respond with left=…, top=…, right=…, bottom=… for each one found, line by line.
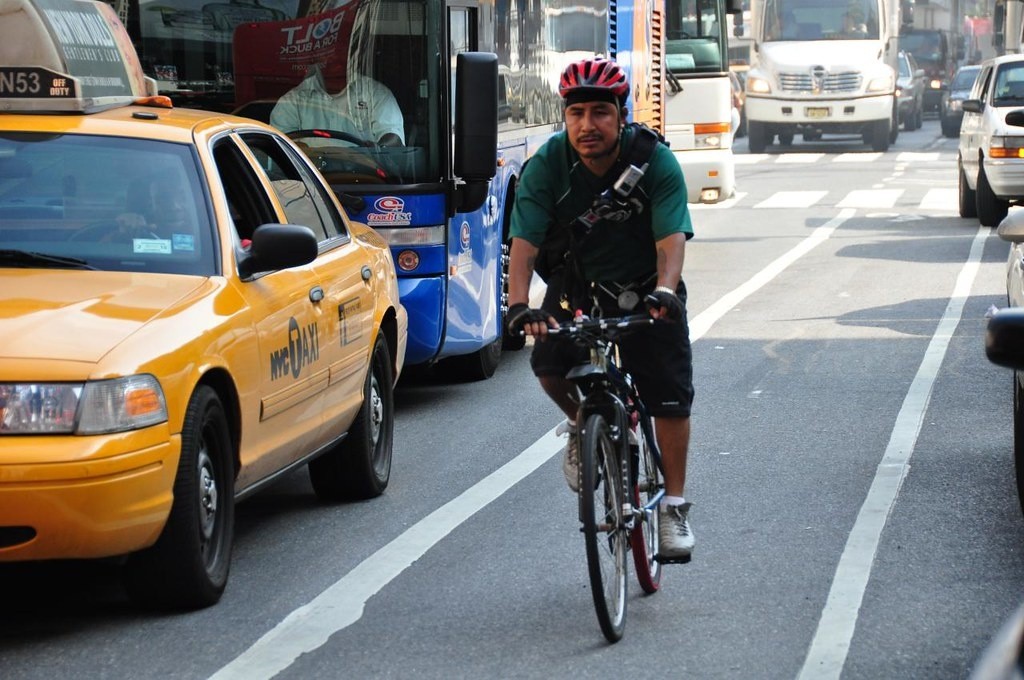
left=99, top=173, right=189, bottom=244
left=269, top=59, right=405, bottom=147
left=507, top=59, right=695, bottom=556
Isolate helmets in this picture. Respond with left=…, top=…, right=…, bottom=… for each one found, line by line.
left=559, top=57, right=629, bottom=102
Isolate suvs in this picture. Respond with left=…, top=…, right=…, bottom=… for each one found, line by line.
left=956, top=54, right=1024, bottom=229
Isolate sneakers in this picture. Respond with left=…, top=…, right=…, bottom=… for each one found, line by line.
left=556, top=420, right=598, bottom=491
left=657, top=497, right=696, bottom=557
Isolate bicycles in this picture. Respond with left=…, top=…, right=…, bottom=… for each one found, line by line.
left=508, top=284, right=692, bottom=642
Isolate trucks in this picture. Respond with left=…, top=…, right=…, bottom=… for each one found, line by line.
left=733, top=0, right=917, bottom=153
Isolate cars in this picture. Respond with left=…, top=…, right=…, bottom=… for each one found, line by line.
left=983, top=207, right=1024, bottom=514
left=0, top=0, right=409, bottom=611
left=894, top=26, right=982, bottom=139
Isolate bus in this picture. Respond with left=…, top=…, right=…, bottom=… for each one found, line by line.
left=95, top=0, right=666, bottom=382
left=666, top=0, right=743, bottom=206
